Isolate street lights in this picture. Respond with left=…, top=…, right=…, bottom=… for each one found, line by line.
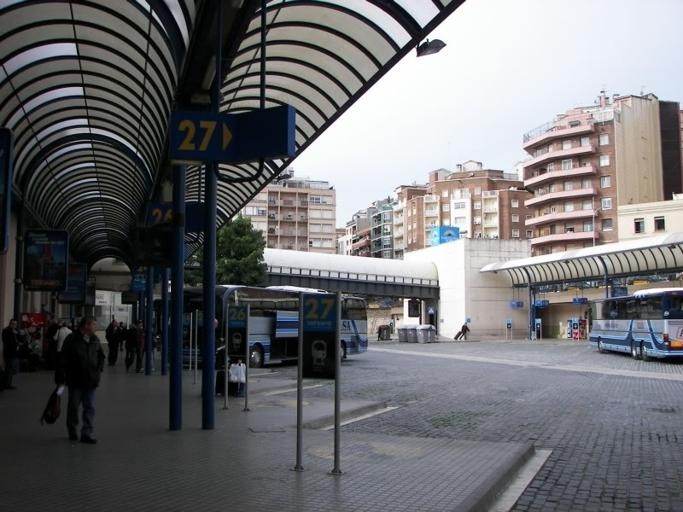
left=593, top=205, right=610, bottom=246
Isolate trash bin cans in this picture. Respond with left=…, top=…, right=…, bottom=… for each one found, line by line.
left=396, top=325, right=436, bottom=344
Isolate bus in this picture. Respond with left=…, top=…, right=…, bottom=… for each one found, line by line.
left=588, top=287, right=683, bottom=362
left=183, top=284, right=368, bottom=368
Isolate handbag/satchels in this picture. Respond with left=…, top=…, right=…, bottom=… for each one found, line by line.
left=41, top=389, right=61, bottom=426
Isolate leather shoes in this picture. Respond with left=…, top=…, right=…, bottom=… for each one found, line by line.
left=70, top=434, right=97, bottom=445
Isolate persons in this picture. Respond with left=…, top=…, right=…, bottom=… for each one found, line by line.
left=426, top=301, right=435, bottom=324
left=376, top=325, right=384, bottom=341
left=459, top=321, right=470, bottom=341
left=52, top=320, right=73, bottom=386
left=104, top=319, right=145, bottom=373
left=54, top=315, right=106, bottom=445
left=2, top=319, right=18, bottom=390
